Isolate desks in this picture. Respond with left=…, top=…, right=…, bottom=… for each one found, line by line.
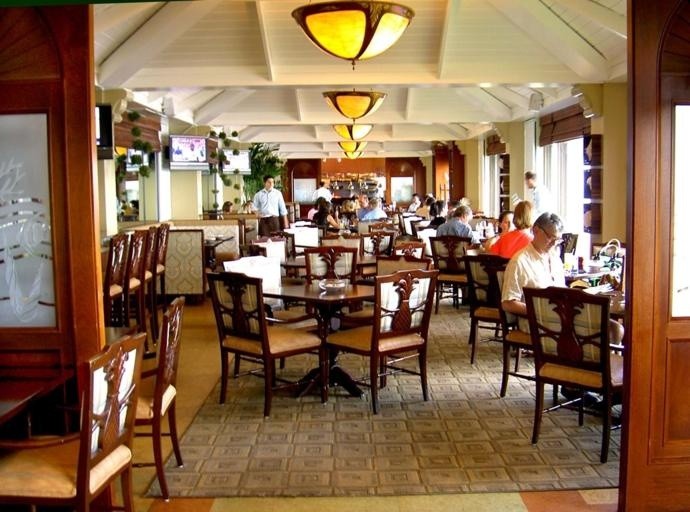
left=259, top=284, right=374, bottom=404
left=0, top=367, right=74, bottom=447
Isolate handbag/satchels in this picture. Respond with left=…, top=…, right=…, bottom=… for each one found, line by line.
left=592, top=238, right=623, bottom=274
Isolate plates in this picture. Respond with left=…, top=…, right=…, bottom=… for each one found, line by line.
left=587, top=265, right=600, bottom=274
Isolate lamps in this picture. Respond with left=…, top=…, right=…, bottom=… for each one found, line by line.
left=333, top=125, right=373, bottom=140
left=344, top=152, right=364, bottom=160
left=321, top=88, right=387, bottom=122
left=290, top=0, right=416, bottom=71
left=337, top=141, right=367, bottom=153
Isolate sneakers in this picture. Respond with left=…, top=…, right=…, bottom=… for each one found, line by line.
left=561, top=385, right=597, bottom=408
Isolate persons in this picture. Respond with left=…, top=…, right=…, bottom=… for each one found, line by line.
left=501, top=213, right=625, bottom=408
left=307, top=181, right=387, bottom=237
left=121, top=200, right=139, bottom=222
left=408, top=171, right=553, bottom=260
left=252, top=176, right=289, bottom=237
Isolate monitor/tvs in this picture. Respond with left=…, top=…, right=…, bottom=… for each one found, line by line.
left=223, top=149, right=248, bottom=175
left=169, top=134, right=210, bottom=170
left=96, top=104, right=115, bottom=161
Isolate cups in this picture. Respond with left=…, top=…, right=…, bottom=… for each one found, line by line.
left=563, top=252, right=579, bottom=274
left=478, top=220, right=487, bottom=237
left=339, top=218, right=349, bottom=228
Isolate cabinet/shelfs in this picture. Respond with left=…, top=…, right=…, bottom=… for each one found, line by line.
left=329, top=178, right=377, bottom=194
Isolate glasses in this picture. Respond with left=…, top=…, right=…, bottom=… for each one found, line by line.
left=538, top=226, right=564, bottom=245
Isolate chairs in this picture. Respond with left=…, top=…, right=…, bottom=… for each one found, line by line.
left=102, top=223, right=171, bottom=344
left=560, top=242, right=624, bottom=323
left=223, top=255, right=315, bottom=369
left=462, top=254, right=521, bottom=372
left=492, top=266, right=558, bottom=406
left=132, top=296, right=186, bottom=499
left=325, top=270, right=438, bottom=414
left=302, top=245, right=358, bottom=312
left=521, top=285, right=625, bottom=464
left=0, top=332, right=148, bottom=512
left=204, top=271, right=328, bottom=416
left=341, top=256, right=433, bottom=329
left=117, top=203, right=499, bottom=316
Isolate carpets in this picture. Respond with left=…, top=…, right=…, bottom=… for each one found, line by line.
left=140, top=282, right=629, bottom=499
